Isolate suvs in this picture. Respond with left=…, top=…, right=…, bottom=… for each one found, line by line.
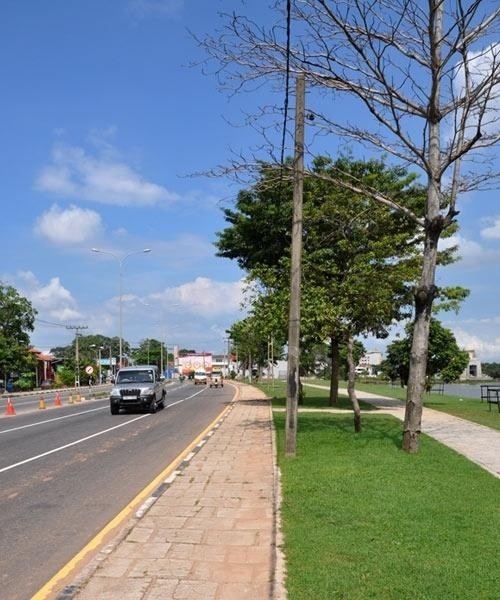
left=109, top=365, right=167, bottom=415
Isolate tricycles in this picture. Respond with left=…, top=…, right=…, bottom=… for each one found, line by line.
left=209, top=372, right=224, bottom=389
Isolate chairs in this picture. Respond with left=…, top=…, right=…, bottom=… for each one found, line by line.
left=481, top=384, right=500, bottom=413
left=428, top=382, right=444, bottom=396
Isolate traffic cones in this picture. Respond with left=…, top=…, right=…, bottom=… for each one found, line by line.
left=54, top=391, right=62, bottom=406
left=4, top=396, right=16, bottom=415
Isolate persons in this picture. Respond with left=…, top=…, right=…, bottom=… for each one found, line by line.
left=141, top=372, right=152, bottom=382
left=178, top=373, right=184, bottom=385
left=88, top=374, right=94, bottom=392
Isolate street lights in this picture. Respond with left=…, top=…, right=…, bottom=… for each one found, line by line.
left=92, top=247, right=151, bottom=368
left=145, top=303, right=180, bottom=380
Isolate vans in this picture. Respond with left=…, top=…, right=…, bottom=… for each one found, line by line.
left=194, top=369, right=207, bottom=385
